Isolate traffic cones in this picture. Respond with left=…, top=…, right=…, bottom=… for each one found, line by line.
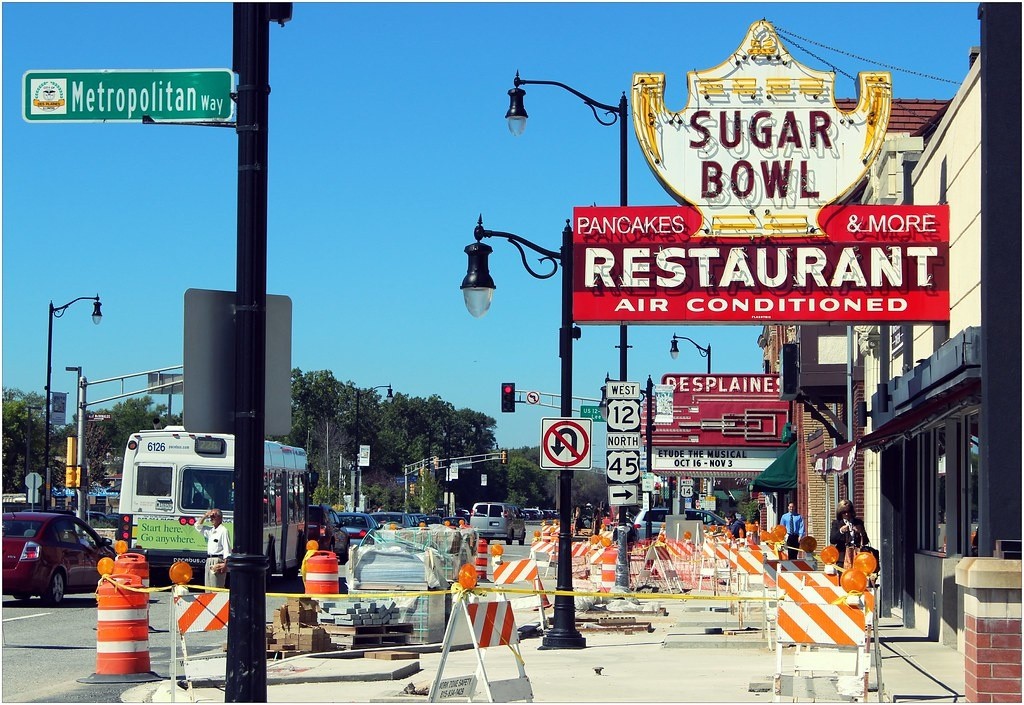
left=533, top=577, right=552, bottom=611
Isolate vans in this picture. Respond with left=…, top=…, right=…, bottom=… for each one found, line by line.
left=470, top=502, right=527, bottom=545
left=633, top=509, right=730, bottom=540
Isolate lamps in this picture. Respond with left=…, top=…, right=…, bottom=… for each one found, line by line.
left=857, top=402, right=873, bottom=429
left=876, top=383, right=892, bottom=414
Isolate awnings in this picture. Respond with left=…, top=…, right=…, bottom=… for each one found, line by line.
left=747, top=441, right=797, bottom=492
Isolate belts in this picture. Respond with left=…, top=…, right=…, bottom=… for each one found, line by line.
left=205, top=554, right=224, bottom=557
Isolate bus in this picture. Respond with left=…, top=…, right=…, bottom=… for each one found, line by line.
left=117, top=425, right=319, bottom=586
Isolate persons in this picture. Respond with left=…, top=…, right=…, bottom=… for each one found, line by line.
left=726, top=512, right=746, bottom=539
left=751, top=505, right=760, bottom=524
left=831, top=499, right=867, bottom=586
left=779, top=501, right=804, bottom=560
left=195, top=509, right=231, bottom=594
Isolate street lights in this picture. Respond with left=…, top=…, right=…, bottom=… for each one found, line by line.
left=42, top=291, right=103, bottom=510
left=670, top=332, right=713, bottom=374
left=353, top=383, right=395, bottom=514
left=64, top=366, right=82, bottom=436
left=598, top=374, right=629, bottom=588
left=460, top=220, right=583, bottom=648
left=505, top=73, right=626, bottom=590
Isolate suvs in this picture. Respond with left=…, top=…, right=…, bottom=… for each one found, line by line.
left=308, top=505, right=350, bottom=563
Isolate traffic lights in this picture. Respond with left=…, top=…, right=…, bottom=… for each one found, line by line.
left=75, top=466, right=89, bottom=488
left=501, top=383, right=515, bottom=413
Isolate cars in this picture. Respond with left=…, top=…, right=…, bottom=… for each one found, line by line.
left=2, top=511, right=117, bottom=604
left=334, top=513, right=381, bottom=544
left=2, top=502, right=119, bottom=536
left=410, top=509, right=470, bottom=529
left=520, top=509, right=558, bottom=519
left=370, top=512, right=414, bottom=544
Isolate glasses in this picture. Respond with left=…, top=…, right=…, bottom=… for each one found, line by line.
left=840, top=510, right=851, bottom=515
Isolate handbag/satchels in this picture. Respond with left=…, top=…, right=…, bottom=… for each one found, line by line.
left=860, top=546, right=880, bottom=573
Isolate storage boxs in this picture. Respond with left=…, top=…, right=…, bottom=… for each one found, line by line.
left=264, top=597, right=330, bottom=655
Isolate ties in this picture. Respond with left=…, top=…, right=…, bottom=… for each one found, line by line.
left=790, top=515, right=794, bottom=536
